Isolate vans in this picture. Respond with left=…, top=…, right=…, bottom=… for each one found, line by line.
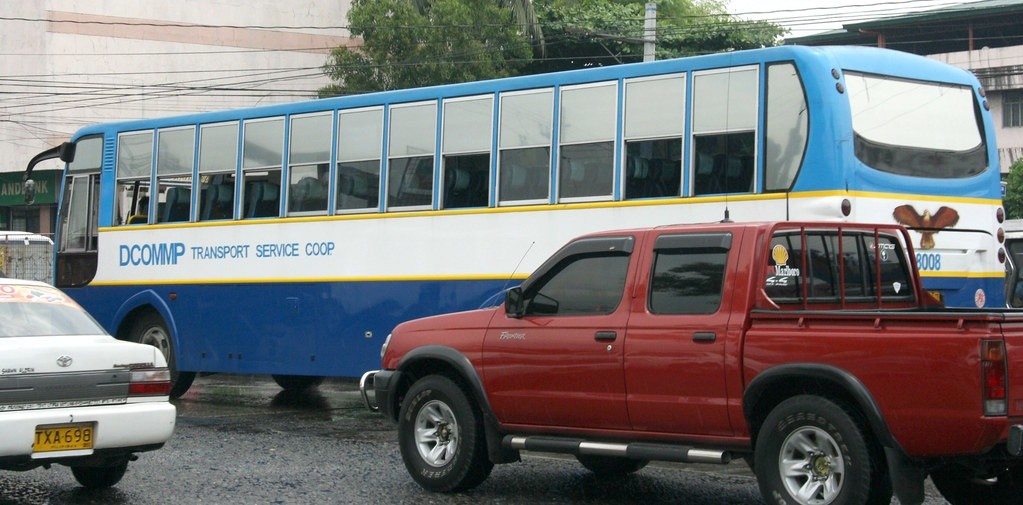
left=0, top=230, right=55, bottom=286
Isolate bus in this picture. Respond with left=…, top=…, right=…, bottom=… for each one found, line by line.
left=22, top=44, right=1010, bottom=399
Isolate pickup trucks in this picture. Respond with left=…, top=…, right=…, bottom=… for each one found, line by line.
left=359, top=221, right=1023, bottom=505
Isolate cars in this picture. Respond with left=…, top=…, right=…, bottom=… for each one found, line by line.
left=0, top=276, right=176, bottom=489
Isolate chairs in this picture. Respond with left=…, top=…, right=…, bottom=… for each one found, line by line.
left=626, top=155, right=653, bottom=200
left=161, top=188, right=192, bottom=222
left=522, top=166, right=550, bottom=199
left=246, top=183, right=280, bottom=217
left=560, top=159, right=587, bottom=197
left=694, top=151, right=723, bottom=195
left=500, top=164, right=531, bottom=200
left=576, top=163, right=611, bottom=197
left=443, top=168, right=471, bottom=208
left=336, top=173, right=372, bottom=209
left=291, top=176, right=322, bottom=212
left=200, top=184, right=235, bottom=220
